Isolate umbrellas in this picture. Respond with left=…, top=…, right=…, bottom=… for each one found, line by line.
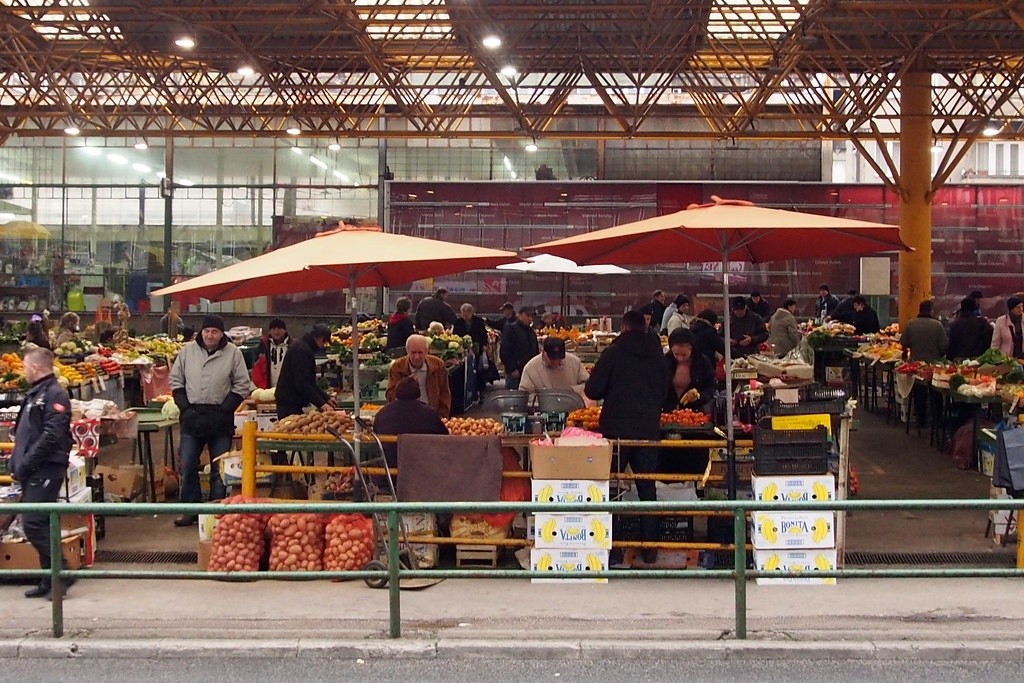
left=149, top=218, right=534, bottom=479
left=496, top=254, right=631, bottom=313
left=521, top=197, right=917, bottom=501
left=0, top=220, right=52, bottom=257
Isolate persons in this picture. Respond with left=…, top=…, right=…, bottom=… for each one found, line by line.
left=717, top=289, right=800, bottom=357
left=500, top=306, right=539, bottom=389
left=10, top=346, right=79, bottom=599
left=586, top=290, right=725, bottom=563
left=453, top=302, right=488, bottom=401
left=253, top=319, right=295, bottom=389
left=386, top=298, right=419, bottom=358
left=899, top=292, right=1024, bottom=360
left=815, top=283, right=879, bottom=333
left=169, top=314, right=251, bottom=527
left=537, top=312, right=553, bottom=331
left=386, top=334, right=451, bottom=419
left=372, top=376, right=450, bottom=468
left=274, top=325, right=337, bottom=420
left=416, top=287, right=457, bottom=330
left=518, top=338, right=589, bottom=391
left=482, top=302, right=517, bottom=386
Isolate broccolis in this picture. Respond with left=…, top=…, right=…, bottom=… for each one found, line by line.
left=51, top=366, right=69, bottom=388
left=360, top=320, right=472, bottom=350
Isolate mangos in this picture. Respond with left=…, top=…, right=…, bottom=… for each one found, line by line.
left=0, top=353, right=25, bottom=378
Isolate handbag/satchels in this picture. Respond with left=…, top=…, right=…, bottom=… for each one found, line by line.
left=476, top=351, right=490, bottom=377
left=992, top=429, right=1024, bottom=490
left=252, top=336, right=270, bottom=389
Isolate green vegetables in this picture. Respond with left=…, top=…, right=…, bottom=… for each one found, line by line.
left=926, top=347, right=1024, bottom=385
left=326, top=340, right=353, bottom=363
left=363, top=353, right=391, bottom=366
left=807, top=330, right=833, bottom=348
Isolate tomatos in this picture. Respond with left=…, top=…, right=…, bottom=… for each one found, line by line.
left=343, top=332, right=362, bottom=347
left=101, top=359, right=122, bottom=374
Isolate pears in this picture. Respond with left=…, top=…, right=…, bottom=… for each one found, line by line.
left=147, top=341, right=178, bottom=355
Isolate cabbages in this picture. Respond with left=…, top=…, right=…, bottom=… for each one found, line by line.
left=53, top=341, right=82, bottom=355
left=162, top=399, right=180, bottom=419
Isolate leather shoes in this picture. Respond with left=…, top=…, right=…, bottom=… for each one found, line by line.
left=48, top=577, right=75, bottom=600
left=25, top=581, right=50, bottom=598
left=174, top=514, right=198, bottom=525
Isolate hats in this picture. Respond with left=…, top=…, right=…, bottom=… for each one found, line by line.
left=1007, top=296, right=1022, bottom=311
left=544, top=337, right=565, bottom=360
left=676, top=298, right=690, bottom=309
left=732, top=296, right=746, bottom=310
left=202, top=315, right=225, bottom=331
left=520, top=305, right=538, bottom=316
left=819, top=284, right=829, bottom=291
left=394, top=377, right=420, bottom=400
left=314, top=323, right=333, bottom=347
left=959, top=295, right=980, bottom=313
left=499, top=302, right=513, bottom=311
left=269, top=319, right=286, bottom=329
left=750, top=288, right=760, bottom=297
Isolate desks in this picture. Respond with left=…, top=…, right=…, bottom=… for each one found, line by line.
left=120, top=408, right=180, bottom=503
left=315, top=359, right=329, bottom=378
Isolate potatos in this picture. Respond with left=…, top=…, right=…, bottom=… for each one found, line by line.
left=849, top=468, right=859, bottom=496
left=566, top=405, right=602, bottom=429
left=659, top=408, right=709, bottom=426
left=439, top=416, right=502, bottom=436
left=207, top=494, right=376, bottom=572
left=321, top=475, right=355, bottom=493
left=265, top=411, right=353, bottom=434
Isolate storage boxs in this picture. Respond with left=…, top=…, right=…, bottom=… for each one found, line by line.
left=894, top=383, right=916, bottom=423
left=95, top=465, right=143, bottom=499
left=57, top=455, right=86, bottom=497
left=531, top=480, right=610, bottom=515
left=359, top=403, right=382, bottom=419
left=534, top=516, right=612, bottom=551
left=931, top=370, right=952, bottom=389
left=825, top=366, right=843, bottom=382
left=570, top=333, right=613, bottom=363
left=844, top=348, right=862, bottom=358
left=377, top=511, right=440, bottom=566
left=747, top=355, right=814, bottom=387
left=236, top=341, right=260, bottom=370
left=198, top=513, right=218, bottom=572
left=700, top=447, right=753, bottom=501
left=212, top=450, right=274, bottom=485
left=882, top=371, right=890, bottom=383
left=613, top=513, right=694, bottom=545
left=198, top=461, right=225, bottom=495
left=233, top=399, right=278, bottom=437
left=977, top=449, right=995, bottom=478
left=530, top=549, right=609, bottom=585
left=751, top=545, right=837, bottom=585
left=146, top=464, right=166, bottom=503
left=706, top=517, right=749, bottom=570
left=989, top=478, right=1024, bottom=571
left=58, top=511, right=97, bottom=555
left=747, top=474, right=835, bottom=503
left=0, top=535, right=82, bottom=570
left=529, top=439, right=614, bottom=479
left=751, top=379, right=850, bottom=476
left=751, top=509, right=835, bottom=549
left=733, top=371, right=758, bottom=380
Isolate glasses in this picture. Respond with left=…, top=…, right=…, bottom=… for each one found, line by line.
left=320, top=337, right=328, bottom=342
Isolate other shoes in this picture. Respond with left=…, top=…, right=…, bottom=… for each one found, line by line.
left=640, top=547, right=658, bottom=563
left=469, top=394, right=478, bottom=402
left=610, top=548, right=624, bottom=564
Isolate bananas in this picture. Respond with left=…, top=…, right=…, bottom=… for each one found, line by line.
left=680, top=388, right=700, bottom=406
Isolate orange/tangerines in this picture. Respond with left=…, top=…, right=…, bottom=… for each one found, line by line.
left=60, top=365, right=83, bottom=383
